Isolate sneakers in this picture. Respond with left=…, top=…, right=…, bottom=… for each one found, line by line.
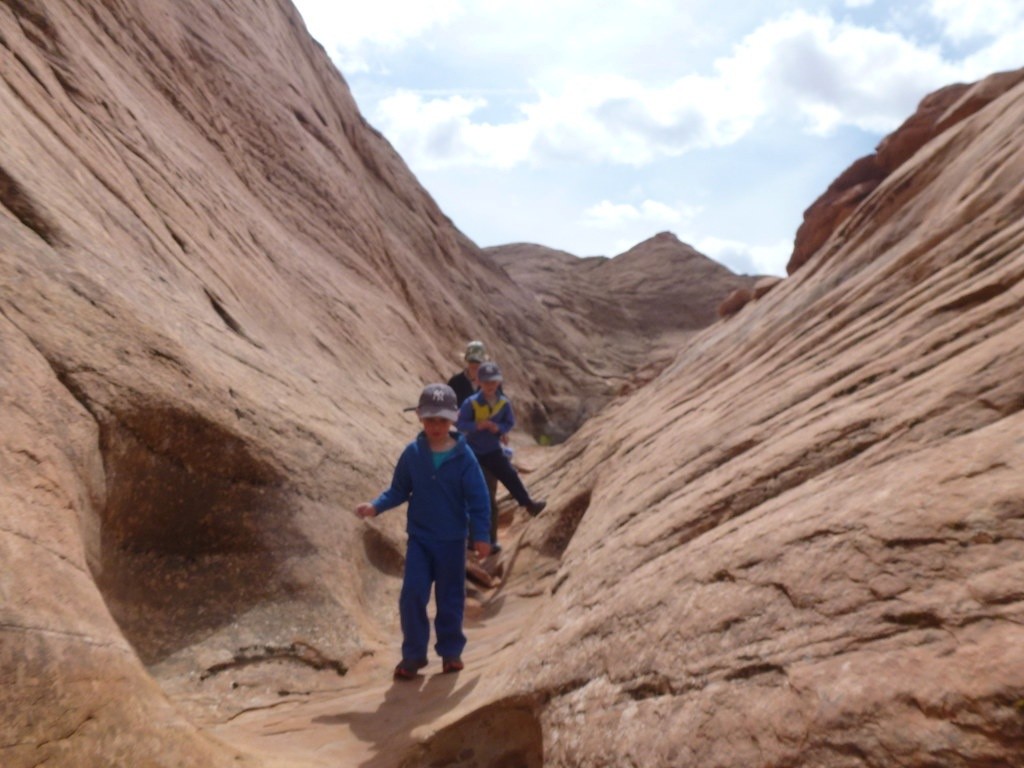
left=393, top=659, right=428, bottom=681
left=442, top=656, right=462, bottom=672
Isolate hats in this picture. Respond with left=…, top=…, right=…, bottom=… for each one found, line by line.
left=416, top=383, right=459, bottom=422
left=464, top=341, right=486, bottom=362
left=477, top=363, right=502, bottom=381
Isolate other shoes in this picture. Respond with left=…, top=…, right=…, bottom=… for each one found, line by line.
left=491, top=543, right=501, bottom=553
left=527, top=501, right=545, bottom=517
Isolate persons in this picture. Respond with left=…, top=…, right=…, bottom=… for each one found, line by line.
left=448, top=340, right=512, bottom=452
left=351, top=382, right=491, bottom=679
left=456, top=362, right=547, bottom=558
